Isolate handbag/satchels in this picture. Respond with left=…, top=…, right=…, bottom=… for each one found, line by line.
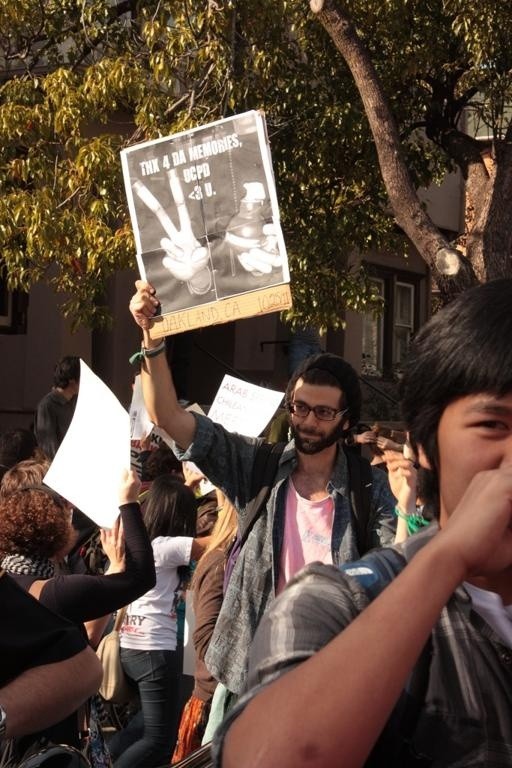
left=96, top=629, right=140, bottom=701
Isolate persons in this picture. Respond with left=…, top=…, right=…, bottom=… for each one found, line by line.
left=210, top=278, right=509, bottom=768
left=130, top=281, right=415, bottom=747
left=0, top=359, right=437, bottom=768
left=133, top=169, right=282, bottom=300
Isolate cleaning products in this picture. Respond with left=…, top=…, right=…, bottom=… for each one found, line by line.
left=222, top=181, right=268, bottom=260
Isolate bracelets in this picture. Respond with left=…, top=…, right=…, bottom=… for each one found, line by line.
left=138, top=337, right=166, bottom=358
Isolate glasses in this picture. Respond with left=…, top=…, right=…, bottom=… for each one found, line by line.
left=287, top=401, right=349, bottom=421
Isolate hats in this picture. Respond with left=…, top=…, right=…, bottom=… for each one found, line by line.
left=284, top=352, right=360, bottom=429
